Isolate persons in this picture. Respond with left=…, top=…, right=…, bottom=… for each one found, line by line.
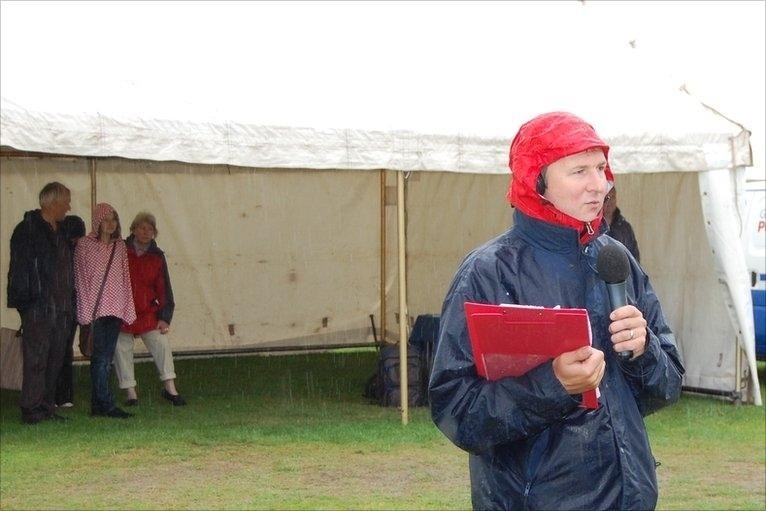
left=114, top=211, right=188, bottom=407
left=603, top=184, right=641, bottom=268
left=6, top=181, right=138, bottom=424
left=427, top=110, right=686, bottom=511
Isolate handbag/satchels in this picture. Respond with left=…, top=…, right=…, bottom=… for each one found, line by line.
left=363, top=343, right=426, bottom=406
left=78, top=325, right=94, bottom=356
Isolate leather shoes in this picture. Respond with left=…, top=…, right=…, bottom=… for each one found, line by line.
left=124, top=397, right=140, bottom=408
left=108, top=406, right=132, bottom=420
left=160, top=385, right=188, bottom=406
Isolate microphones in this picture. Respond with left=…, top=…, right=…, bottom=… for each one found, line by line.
left=596, top=243, right=633, bottom=360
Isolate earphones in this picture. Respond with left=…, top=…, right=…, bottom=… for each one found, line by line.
left=536, top=174, right=545, bottom=196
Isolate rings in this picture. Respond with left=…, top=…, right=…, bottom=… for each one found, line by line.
left=164, top=328, right=168, bottom=331
left=630, top=328, right=634, bottom=339
